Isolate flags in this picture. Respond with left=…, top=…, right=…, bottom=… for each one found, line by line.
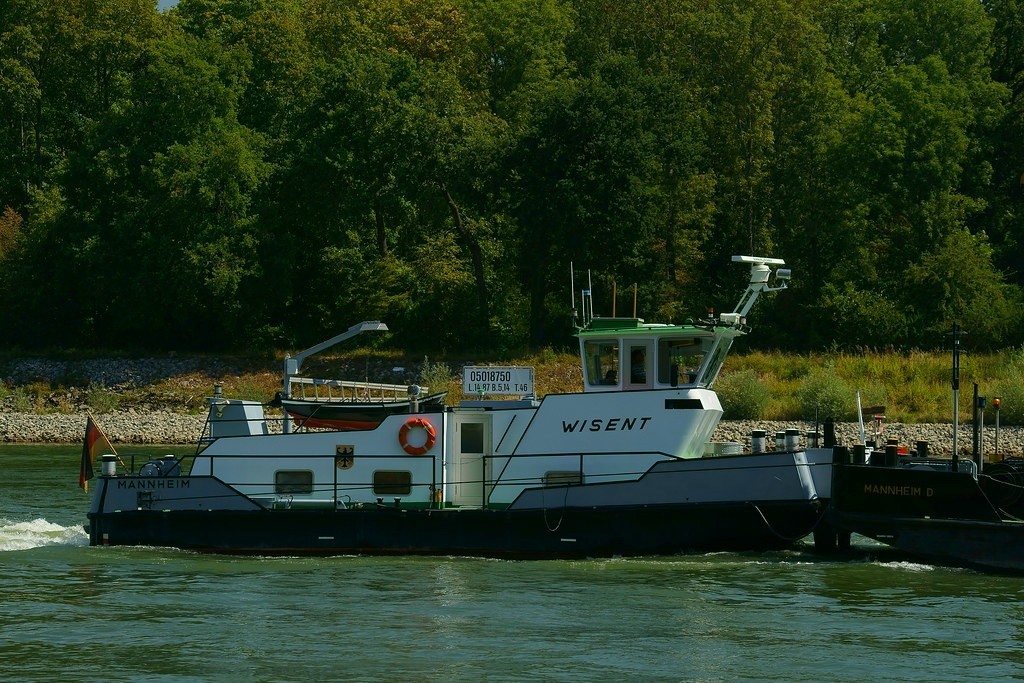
left=79, top=416, right=110, bottom=495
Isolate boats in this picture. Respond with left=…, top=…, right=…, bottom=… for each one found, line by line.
left=279, top=375, right=450, bottom=430
left=81, top=253, right=872, bottom=576
left=822, top=319, right=1024, bottom=570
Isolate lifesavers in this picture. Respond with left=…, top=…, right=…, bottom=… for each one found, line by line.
left=398, top=417, right=437, bottom=455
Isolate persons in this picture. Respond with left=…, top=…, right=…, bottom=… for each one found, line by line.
left=600, top=370, right=618, bottom=385
left=631, top=349, right=645, bottom=384
